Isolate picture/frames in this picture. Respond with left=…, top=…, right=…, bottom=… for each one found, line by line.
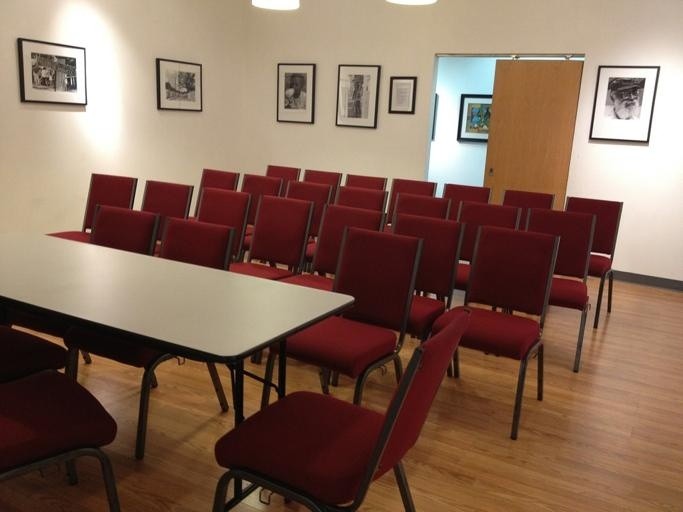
left=332, top=62, right=383, bottom=131
left=15, top=35, right=90, bottom=110
left=587, top=62, right=661, bottom=149
left=153, top=56, right=204, bottom=115
left=429, top=91, right=440, bottom=140
left=273, top=62, right=317, bottom=125
left=387, top=75, right=417, bottom=118
left=454, top=92, right=492, bottom=147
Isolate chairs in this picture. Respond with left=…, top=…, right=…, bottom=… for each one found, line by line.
left=44, top=165, right=623, bottom=440
left=212, top=309, right=470, bottom=512
left=1, top=326, right=120, bottom=512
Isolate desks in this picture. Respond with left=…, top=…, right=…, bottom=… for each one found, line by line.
left=1, top=231, right=355, bottom=498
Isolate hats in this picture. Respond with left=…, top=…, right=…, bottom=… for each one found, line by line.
left=607, top=77, right=641, bottom=91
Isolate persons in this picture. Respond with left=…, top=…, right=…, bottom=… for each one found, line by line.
left=285, top=74, right=306, bottom=109
left=41, top=67, right=50, bottom=84
left=604, top=78, right=645, bottom=120
left=470, top=107, right=490, bottom=129
